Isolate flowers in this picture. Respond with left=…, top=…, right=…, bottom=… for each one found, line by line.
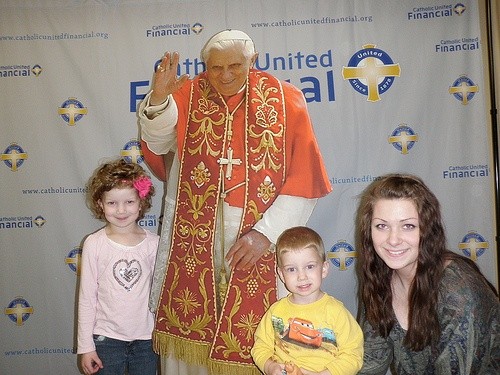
left=133, top=177, right=152, bottom=200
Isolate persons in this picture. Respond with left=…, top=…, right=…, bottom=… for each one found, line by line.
left=356, top=174, right=500, bottom=375
left=138, top=29, right=333, bottom=375
left=250, top=227, right=365, bottom=375
left=76, top=159, right=160, bottom=375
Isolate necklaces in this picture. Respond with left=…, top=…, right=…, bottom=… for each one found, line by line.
left=217, top=91, right=245, bottom=180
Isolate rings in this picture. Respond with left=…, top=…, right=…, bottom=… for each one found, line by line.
left=157, top=65, right=165, bottom=72
